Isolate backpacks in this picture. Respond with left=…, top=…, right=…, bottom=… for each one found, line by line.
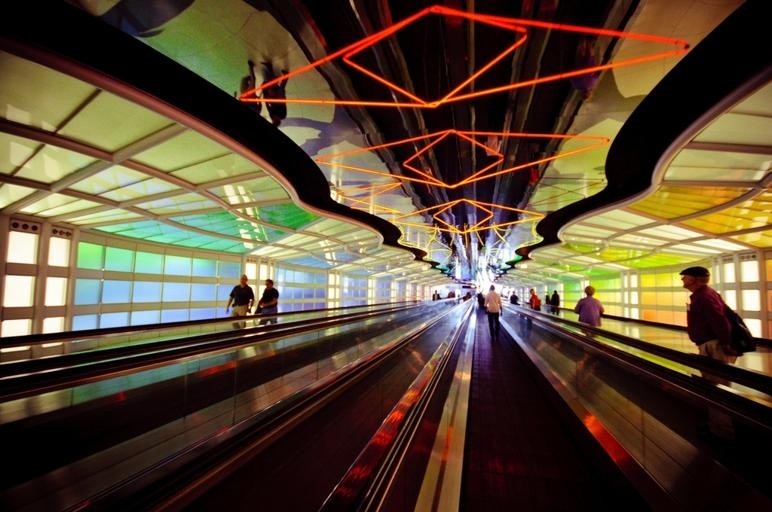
left=720, top=301, right=757, bottom=357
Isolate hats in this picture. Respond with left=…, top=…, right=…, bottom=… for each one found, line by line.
left=678, top=265, right=711, bottom=278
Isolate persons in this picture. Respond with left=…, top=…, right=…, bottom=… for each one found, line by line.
left=447, top=290, right=485, bottom=308
left=510, top=291, right=521, bottom=305
left=233, top=60, right=290, bottom=129
left=545, top=290, right=560, bottom=316
left=226, top=274, right=279, bottom=330
left=529, top=288, right=541, bottom=311
left=679, top=266, right=743, bottom=388
left=484, top=285, right=503, bottom=341
left=574, top=286, right=605, bottom=327
left=432, top=290, right=440, bottom=301
